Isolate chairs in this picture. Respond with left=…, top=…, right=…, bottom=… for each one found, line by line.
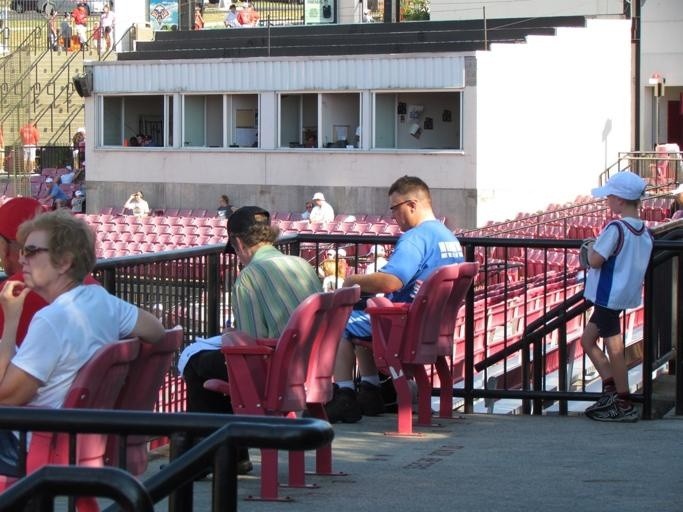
left=204, top=283, right=361, bottom=503
left=3, top=190, right=411, bottom=314
left=2, top=323, right=185, bottom=512
left=440, top=182, right=680, bottom=327
left=353, top=262, right=479, bottom=434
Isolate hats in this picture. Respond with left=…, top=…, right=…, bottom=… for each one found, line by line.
left=44, top=177, right=52, bottom=183
left=590, top=172, right=649, bottom=200
left=311, top=192, right=325, bottom=201
left=223, top=206, right=271, bottom=254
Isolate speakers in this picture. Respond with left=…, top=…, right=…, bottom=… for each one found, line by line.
left=73, top=73, right=91, bottom=97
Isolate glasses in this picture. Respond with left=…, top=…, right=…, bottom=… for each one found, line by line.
left=18, top=244, right=50, bottom=257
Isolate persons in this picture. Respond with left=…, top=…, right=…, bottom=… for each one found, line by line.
left=305, top=134, right=316, bottom=147
left=355, top=126, right=360, bottom=147
left=301, top=201, right=313, bottom=219
left=326, top=175, right=465, bottom=423
left=321, top=244, right=388, bottom=290
left=129, top=134, right=152, bottom=146
left=310, top=192, right=335, bottom=224
left=48, top=3, right=115, bottom=58
left=124, top=191, right=149, bottom=216
left=160, top=206, right=324, bottom=479
left=215, top=195, right=238, bottom=219
left=668, top=182, right=683, bottom=220
left=0, top=127, right=8, bottom=173
left=252, top=133, right=258, bottom=147
left=195, top=6, right=204, bottom=30
left=1, top=206, right=167, bottom=477
left=45, top=130, right=87, bottom=214
left=19, top=120, right=41, bottom=172
left=580, top=173, right=654, bottom=422
left=1, top=197, right=101, bottom=352
left=225, top=3, right=260, bottom=28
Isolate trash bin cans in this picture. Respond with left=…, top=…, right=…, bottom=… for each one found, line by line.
left=5, top=145, right=24, bottom=173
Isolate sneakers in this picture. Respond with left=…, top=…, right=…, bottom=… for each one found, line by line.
left=585, top=384, right=643, bottom=422
left=303, top=380, right=385, bottom=423
